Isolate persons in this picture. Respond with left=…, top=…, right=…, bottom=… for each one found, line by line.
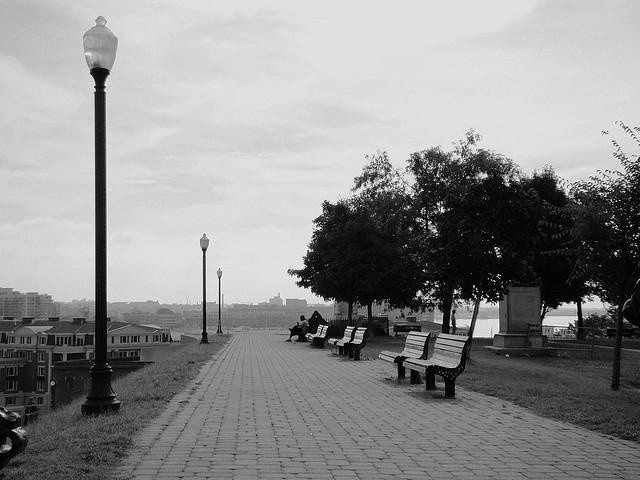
left=287, top=315, right=307, bottom=342
left=451, top=310, right=456, bottom=334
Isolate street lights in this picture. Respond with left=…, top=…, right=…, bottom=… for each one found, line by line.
left=216, top=267, right=222, bottom=333
left=82, top=15, right=121, bottom=417
left=200, top=232, right=210, bottom=345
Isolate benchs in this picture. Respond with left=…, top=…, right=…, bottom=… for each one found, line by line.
left=308, top=326, right=328, bottom=349
left=403, top=333, right=474, bottom=399
left=336, top=327, right=367, bottom=361
left=305, top=324, right=323, bottom=347
left=379, top=331, right=431, bottom=385
left=392, top=324, right=422, bottom=335
left=328, top=326, right=355, bottom=355
